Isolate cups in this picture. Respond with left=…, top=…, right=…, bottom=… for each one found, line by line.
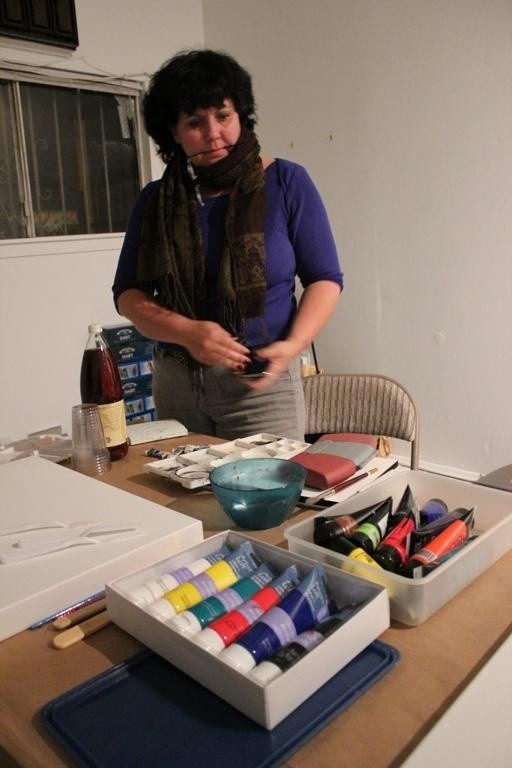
left=69, top=403, right=111, bottom=477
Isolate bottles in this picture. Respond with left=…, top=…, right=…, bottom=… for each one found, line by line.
left=79, top=323, right=128, bottom=462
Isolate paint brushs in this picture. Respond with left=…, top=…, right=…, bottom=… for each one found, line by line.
left=304, top=466, right=378, bottom=512
left=29, top=590, right=106, bottom=633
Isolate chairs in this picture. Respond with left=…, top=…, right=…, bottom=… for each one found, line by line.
left=302, top=373, right=419, bottom=471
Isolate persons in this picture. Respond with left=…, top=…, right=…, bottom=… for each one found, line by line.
left=113, top=48, right=345, bottom=447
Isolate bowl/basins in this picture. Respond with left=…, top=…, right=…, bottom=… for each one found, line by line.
left=208, top=457, right=309, bottom=531
